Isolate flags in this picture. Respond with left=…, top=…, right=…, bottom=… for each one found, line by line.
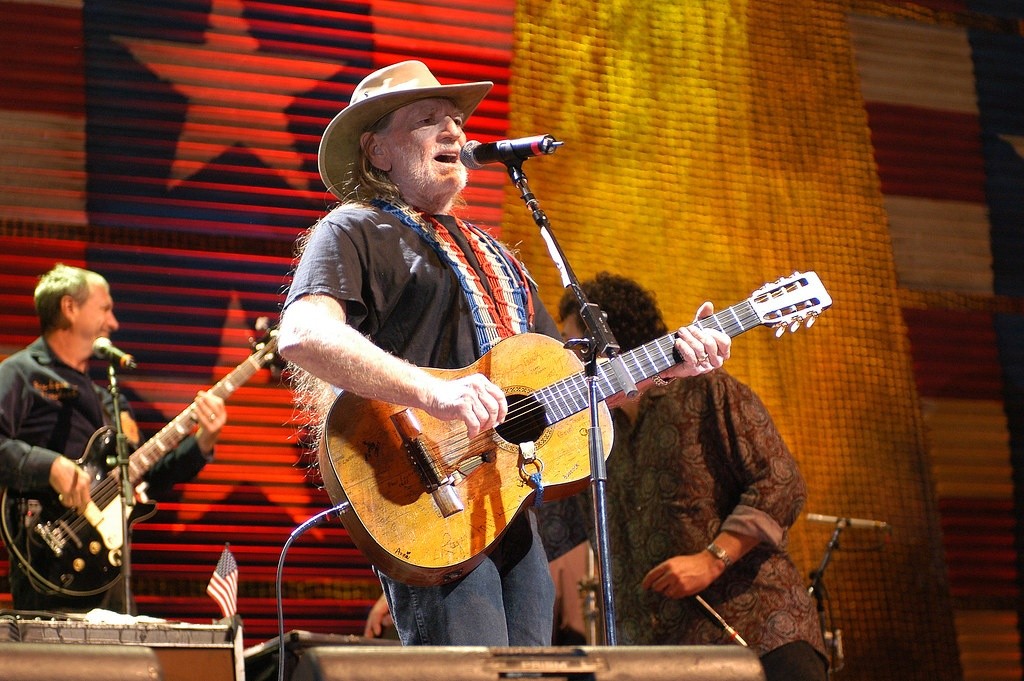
left=206, top=548, right=238, bottom=617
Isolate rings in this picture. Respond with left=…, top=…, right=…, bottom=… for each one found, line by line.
left=210, top=413, right=216, bottom=421
left=697, top=354, right=708, bottom=362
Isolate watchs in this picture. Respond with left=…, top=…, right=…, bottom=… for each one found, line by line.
left=651, top=373, right=677, bottom=388
left=707, top=543, right=733, bottom=569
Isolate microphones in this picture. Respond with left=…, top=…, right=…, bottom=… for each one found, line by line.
left=807, top=513, right=890, bottom=531
left=693, top=594, right=749, bottom=649
left=461, top=133, right=564, bottom=169
left=92, top=337, right=137, bottom=369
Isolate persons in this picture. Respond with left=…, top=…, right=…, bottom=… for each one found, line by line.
left=276, top=60, right=735, bottom=648
left=0, top=264, right=227, bottom=617
left=360, top=271, right=830, bottom=681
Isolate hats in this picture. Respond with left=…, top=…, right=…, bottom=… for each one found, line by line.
left=317, top=59, right=494, bottom=202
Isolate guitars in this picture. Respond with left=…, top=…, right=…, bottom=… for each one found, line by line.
left=0, top=315, right=279, bottom=601
left=316, top=267, right=834, bottom=586
